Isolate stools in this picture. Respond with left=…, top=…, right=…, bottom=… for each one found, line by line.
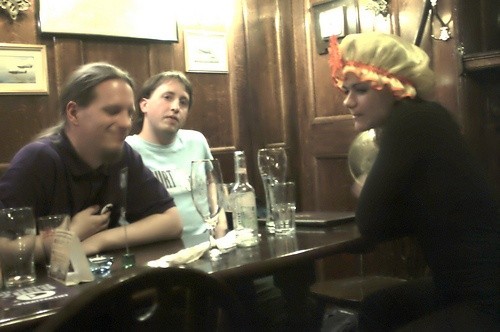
left=308, top=272, right=410, bottom=332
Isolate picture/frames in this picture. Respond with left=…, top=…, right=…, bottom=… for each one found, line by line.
left=184, top=29, right=228, bottom=73
left=0, top=43, right=49, bottom=94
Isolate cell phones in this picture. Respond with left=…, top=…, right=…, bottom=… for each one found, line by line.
left=99, top=203, right=113, bottom=215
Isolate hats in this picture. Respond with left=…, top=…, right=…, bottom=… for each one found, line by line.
left=327, top=31, right=431, bottom=98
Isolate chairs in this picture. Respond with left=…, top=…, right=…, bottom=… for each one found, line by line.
left=34, top=264, right=258, bottom=331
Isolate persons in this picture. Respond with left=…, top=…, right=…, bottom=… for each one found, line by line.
left=126, top=70, right=228, bottom=231
left=0, top=62, right=184, bottom=277
left=330, top=34, right=500, bottom=332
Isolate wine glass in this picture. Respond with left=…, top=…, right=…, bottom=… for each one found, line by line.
left=191, top=159, right=225, bottom=258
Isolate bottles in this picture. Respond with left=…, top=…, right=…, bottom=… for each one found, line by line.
left=228, top=151, right=258, bottom=248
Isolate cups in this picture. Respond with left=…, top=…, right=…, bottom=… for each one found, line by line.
left=88, top=251, right=114, bottom=278
left=257, top=148, right=287, bottom=234
left=267, top=182, right=297, bottom=238
left=38, top=212, right=73, bottom=281
left=0, top=207, right=36, bottom=289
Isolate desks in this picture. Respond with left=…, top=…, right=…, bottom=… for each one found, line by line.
left=0, top=227, right=363, bottom=332
left=227, top=208, right=366, bottom=275
